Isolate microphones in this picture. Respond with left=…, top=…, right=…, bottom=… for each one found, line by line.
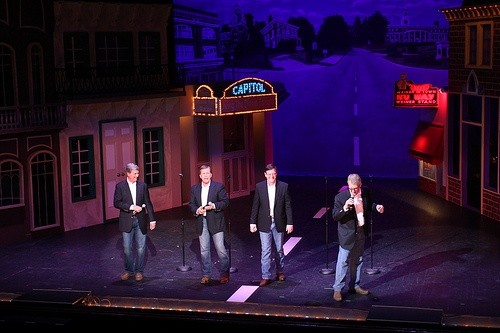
left=202, top=202, right=212, bottom=209
left=133, top=204, right=146, bottom=215
left=349, top=195, right=355, bottom=211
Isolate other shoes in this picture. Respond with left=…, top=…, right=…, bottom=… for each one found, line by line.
left=349, top=287, right=369, bottom=295
left=333, top=291, right=343, bottom=302
left=135, top=273, right=143, bottom=281
left=122, top=272, right=134, bottom=281
left=200, top=276, right=212, bottom=284
left=259, top=279, right=271, bottom=286
left=276, top=273, right=286, bottom=281
left=220, top=275, right=230, bottom=284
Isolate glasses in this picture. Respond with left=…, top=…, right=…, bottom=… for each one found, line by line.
left=266, top=172, right=277, bottom=176
left=348, top=188, right=360, bottom=191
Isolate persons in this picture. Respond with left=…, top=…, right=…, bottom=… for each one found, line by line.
left=332, top=173, right=384, bottom=304
left=250, top=164, right=293, bottom=286
left=189, top=163, right=230, bottom=284
left=114, top=163, right=156, bottom=281
